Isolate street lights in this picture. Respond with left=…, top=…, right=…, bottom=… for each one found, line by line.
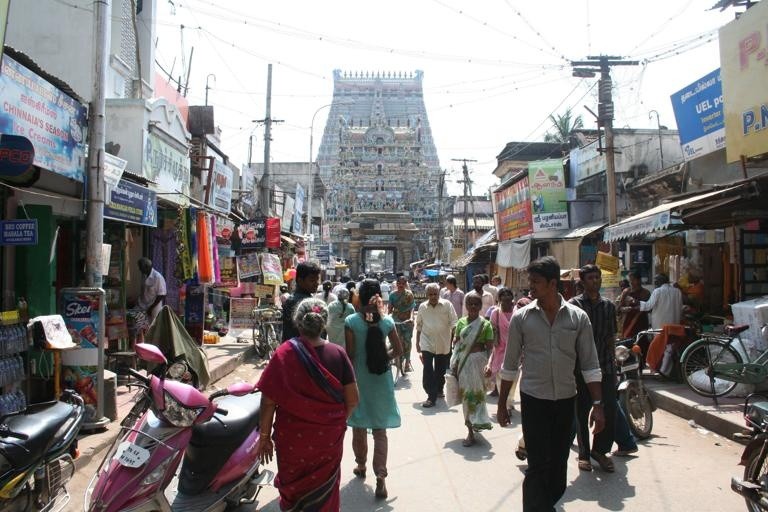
left=308, top=99, right=355, bottom=235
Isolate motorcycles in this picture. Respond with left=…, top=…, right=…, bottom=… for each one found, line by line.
left=120, top=303, right=211, bottom=432
left=88, top=343, right=274, bottom=511
left=613, top=329, right=663, bottom=440
left=0, top=390, right=86, bottom=512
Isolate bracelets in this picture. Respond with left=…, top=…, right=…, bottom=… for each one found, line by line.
left=259, top=432, right=270, bottom=439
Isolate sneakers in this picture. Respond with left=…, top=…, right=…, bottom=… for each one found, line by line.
left=352, top=364, right=638, bottom=499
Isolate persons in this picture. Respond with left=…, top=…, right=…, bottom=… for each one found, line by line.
left=345, top=277, right=404, bottom=498
left=566, top=264, right=638, bottom=473
left=254, top=297, right=362, bottom=512
left=613, top=266, right=705, bottom=367
left=380, top=273, right=534, bottom=449
left=495, top=255, right=606, bottom=510
left=134, top=256, right=167, bottom=320
left=278, top=259, right=365, bottom=347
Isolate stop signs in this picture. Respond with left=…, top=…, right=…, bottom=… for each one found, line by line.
left=247, top=230, right=255, bottom=240
left=221, top=228, right=231, bottom=239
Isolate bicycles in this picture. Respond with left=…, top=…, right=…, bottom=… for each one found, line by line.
left=387, top=319, right=413, bottom=387
left=678, top=321, right=767, bottom=405
left=248, top=308, right=278, bottom=360
left=729, top=392, right=768, bottom=512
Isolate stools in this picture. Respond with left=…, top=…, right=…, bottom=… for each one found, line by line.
left=724, top=323, right=749, bottom=338
left=106, top=351, right=142, bottom=393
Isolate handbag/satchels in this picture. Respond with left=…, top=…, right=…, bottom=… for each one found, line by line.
left=487, top=326, right=499, bottom=346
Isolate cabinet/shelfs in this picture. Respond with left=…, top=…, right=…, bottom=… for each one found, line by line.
left=102, top=239, right=126, bottom=352
left=625, top=242, right=655, bottom=291
left=723, top=228, right=768, bottom=303
left=1, top=319, right=31, bottom=419
